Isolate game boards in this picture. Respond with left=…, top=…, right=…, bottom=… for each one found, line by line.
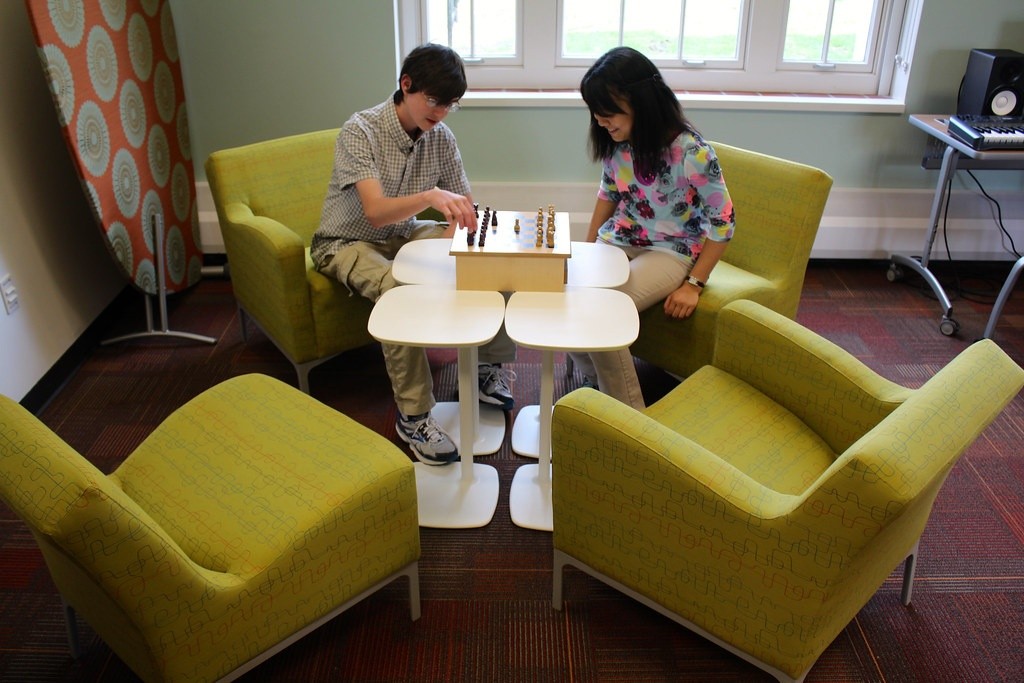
left=450, top=211, right=571, bottom=255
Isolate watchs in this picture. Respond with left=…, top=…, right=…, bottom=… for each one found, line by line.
left=685, top=275, right=704, bottom=288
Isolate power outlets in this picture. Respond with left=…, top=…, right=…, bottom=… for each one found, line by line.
left=0, top=273, right=20, bottom=314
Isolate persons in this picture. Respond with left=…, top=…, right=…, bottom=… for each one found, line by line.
left=312, top=47, right=513, bottom=466
left=579, top=46, right=737, bottom=409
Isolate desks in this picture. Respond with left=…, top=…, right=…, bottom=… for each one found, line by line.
left=504, top=287, right=640, bottom=531
left=883, top=111, right=1024, bottom=337
left=391, top=237, right=506, bottom=456
left=511, top=241, right=631, bottom=459
left=367, top=285, right=505, bottom=529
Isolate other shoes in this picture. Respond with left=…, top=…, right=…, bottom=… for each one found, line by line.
left=583, top=376, right=599, bottom=391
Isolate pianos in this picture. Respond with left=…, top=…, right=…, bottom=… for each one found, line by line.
left=947, top=112, right=1024, bottom=151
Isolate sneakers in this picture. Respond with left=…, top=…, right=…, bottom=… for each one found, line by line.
left=456, top=361, right=517, bottom=409
left=396, top=411, right=458, bottom=466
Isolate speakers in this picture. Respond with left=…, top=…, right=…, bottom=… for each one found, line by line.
left=957, top=49, right=1024, bottom=120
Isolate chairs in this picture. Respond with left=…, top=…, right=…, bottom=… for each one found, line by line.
left=566, top=138, right=834, bottom=382
left=0, top=371, right=421, bottom=683
left=550, top=298, right=1024, bottom=683
left=204, top=127, right=377, bottom=397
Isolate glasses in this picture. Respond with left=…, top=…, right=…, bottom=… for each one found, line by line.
left=416, top=89, right=462, bottom=115
